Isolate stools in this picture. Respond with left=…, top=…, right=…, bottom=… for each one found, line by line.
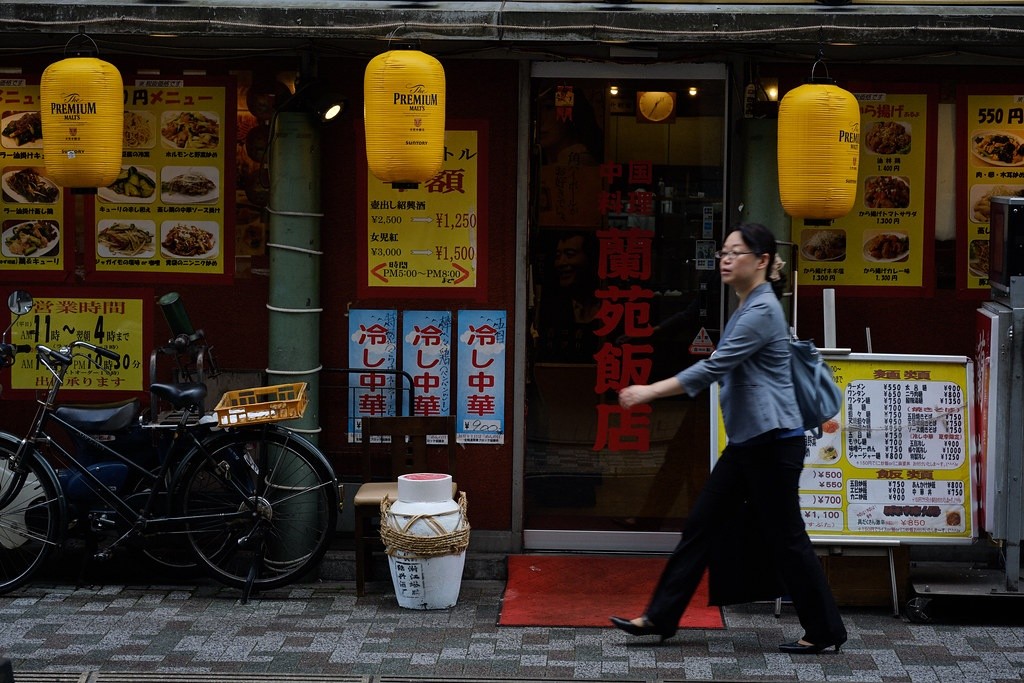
left=354, top=482, right=457, bottom=597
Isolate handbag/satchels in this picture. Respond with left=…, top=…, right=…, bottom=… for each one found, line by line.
left=787, top=328, right=842, bottom=439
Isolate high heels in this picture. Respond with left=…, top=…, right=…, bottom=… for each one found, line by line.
left=778, top=637, right=841, bottom=656
left=611, top=616, right=676, bottom=643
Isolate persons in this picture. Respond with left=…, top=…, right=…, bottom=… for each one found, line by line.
left=610, top=222, right=849, bottom=654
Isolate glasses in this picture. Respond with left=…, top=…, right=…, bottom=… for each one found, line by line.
left=714, top=250, right=753, bottom=259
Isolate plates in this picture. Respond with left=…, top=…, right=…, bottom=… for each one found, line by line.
left=0, top=112, right=44, bottom=149
left=161, top=221, right=220, bottom=259
left=1, top=221, right=60, bottom=258
left=161, top=110, right=219, bottom=149
left=969, top=185, right=1023, bottom=224
left=161, top=166, right=219, bottom=202
left=98, top=164, right=156, bottom=204
left=863, top=232, right=911, bottom=263
left=971, top=130, right=1024, bottom=167
left=801, top=240, right=846, bottom=261
left=98, top=220, right=155, bottom=258
left=1, top=170, right=60, bottom=203
